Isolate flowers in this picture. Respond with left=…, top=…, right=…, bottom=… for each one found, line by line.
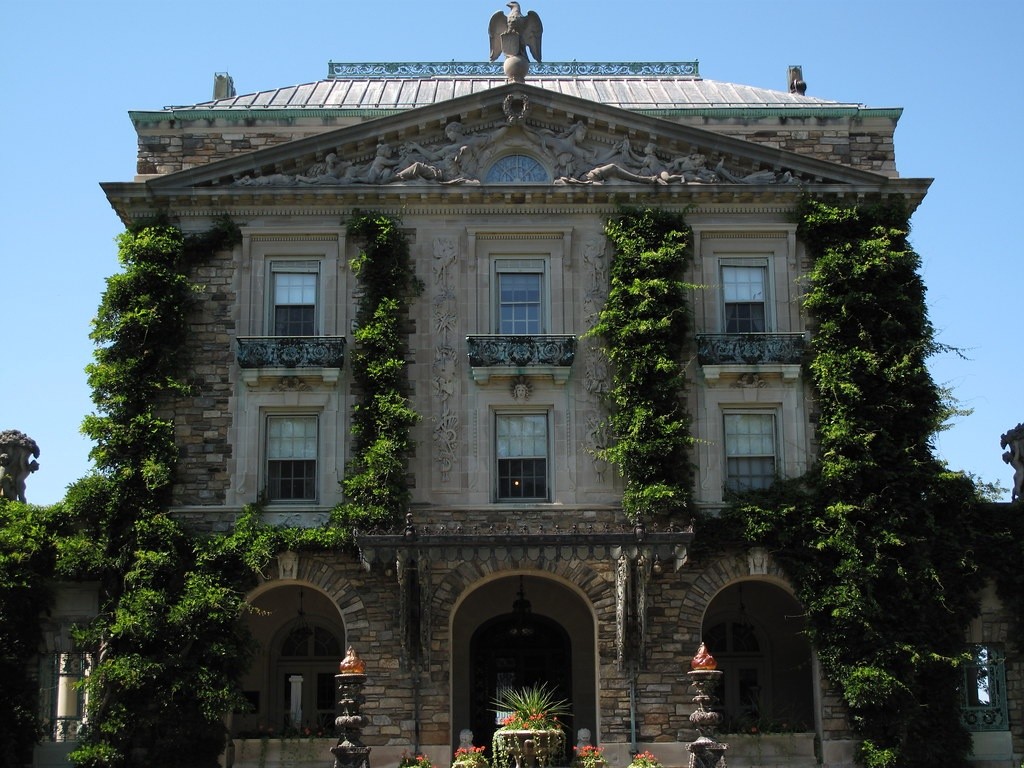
left=492, top=682, right=576, bottom=732
left=632, top=751, right=662, bottom=767
left=401, top=748, right=433, bottom=767
left=572, top=746, right=609, bottom=767
left=454, top=746, right=488, bottom=766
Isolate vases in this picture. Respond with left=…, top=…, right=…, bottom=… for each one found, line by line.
left=453, top=762, right=482, bottom=768
left=577, top=760, right=604, bottom=768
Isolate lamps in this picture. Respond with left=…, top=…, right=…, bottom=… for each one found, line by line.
left=357, top=548, right=371, bottom=574
left=653, top=553, right=665, bottom=575
